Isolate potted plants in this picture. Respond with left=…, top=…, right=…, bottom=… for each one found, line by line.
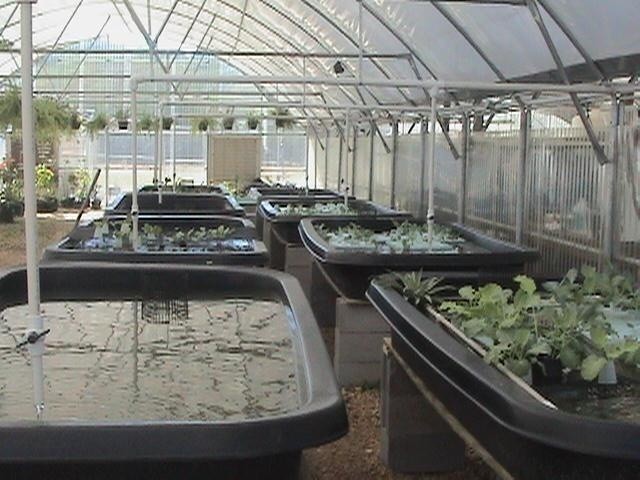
left=245, top=110, right=261, bottom=130
left=266, top=105, right=292, bottom=128
left=162, top=115, right=174, bottom=131
left=221, top=111, right=236, bottom=130
left=64, top=110, right=84, bottom=130
left=112, top=109, right=131, bottom=131
left=191, top=116, right=218, bottom=133
